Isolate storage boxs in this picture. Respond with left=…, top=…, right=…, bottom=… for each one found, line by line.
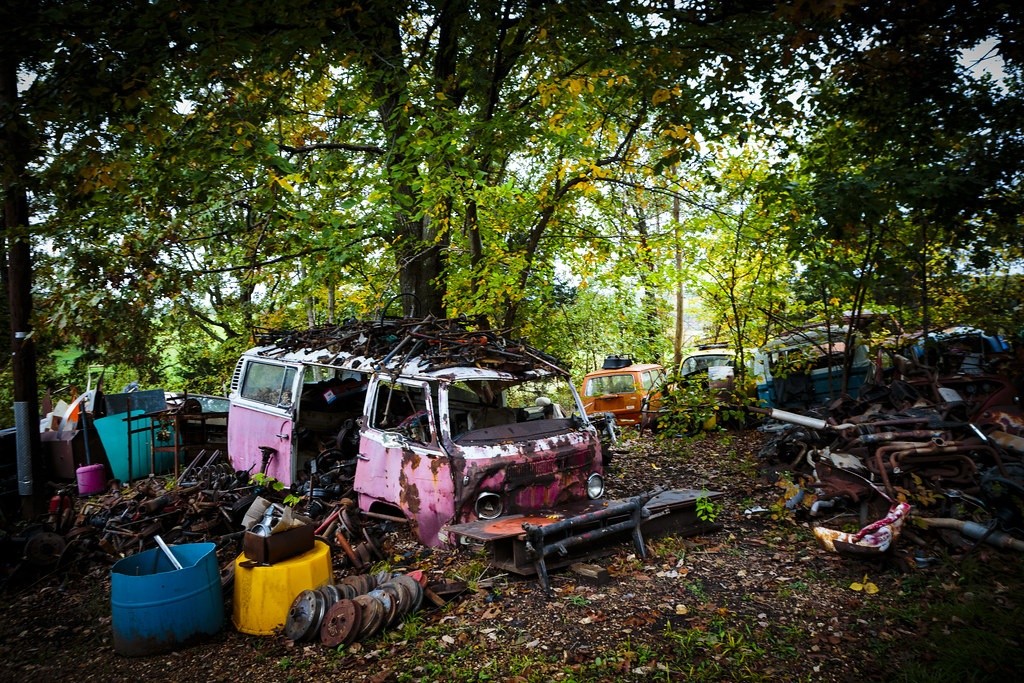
left=244, top=513, right=315, bottom=561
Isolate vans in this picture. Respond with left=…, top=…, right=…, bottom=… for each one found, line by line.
left=662, top=305, right=1018, bottom=455
left=578, top=354, right=671, bottom=431
left=227, top=344, right=606, bottom=557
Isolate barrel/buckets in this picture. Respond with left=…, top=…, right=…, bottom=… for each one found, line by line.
left=110, top=542, right=226, bottom=657
left=76, top=464, right=106, bottom=494
